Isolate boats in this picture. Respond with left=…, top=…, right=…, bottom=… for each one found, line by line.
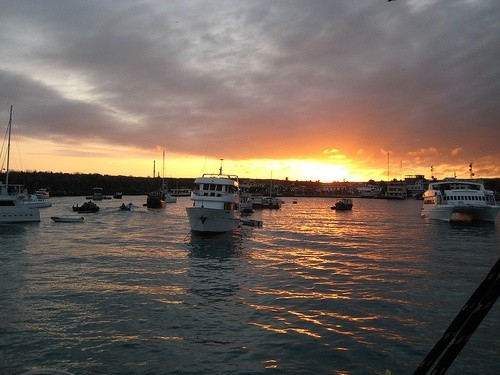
left=331, top=194, right=353, bottom=211
left=113, top=191, right=123, bottom=200
left=0, top=196, right=42, bottom=225
left=50, top=216, right=86, bottom=224
left=142, top=150, right=178, bottom=208
left=419, top=177, right=500, bottom=223
left=92, top=185, right=105, bottom=201
left=72, top=199, right=100, bottom=213
left=185, top=159, right=285, bottom=236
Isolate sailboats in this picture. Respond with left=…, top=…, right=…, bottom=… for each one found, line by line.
left=0, top=105, right=53, bottom=208
